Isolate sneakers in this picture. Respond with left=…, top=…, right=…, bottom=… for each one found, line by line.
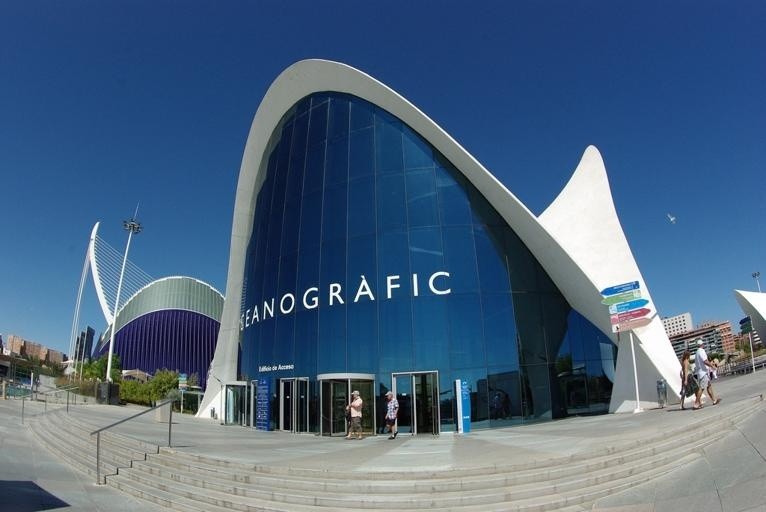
left=680, top=398, right=722, bottom=409
left=344, top=436, right=363, bottom=440
left=388, top=430, right=398, bottom=439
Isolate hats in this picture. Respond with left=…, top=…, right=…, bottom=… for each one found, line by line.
left=351, top=390, right=359, bottom=395
left=385, top=391, right=394, bottom=396
left=697, top=339, right=706, bottom=346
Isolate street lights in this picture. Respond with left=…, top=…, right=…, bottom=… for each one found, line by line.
left=104, top=199, right=145, bottom=382
left=752, top=270, right=762, bottom=294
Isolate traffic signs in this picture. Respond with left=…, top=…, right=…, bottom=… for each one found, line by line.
left=739, top=315, right=753, bottom=334
left=600, top=279, right=653, bottom=334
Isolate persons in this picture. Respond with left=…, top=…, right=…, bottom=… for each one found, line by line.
left=384, top=390, right=400, bottom=439
left=675, top=351, right=705, bottom=410
left=499, top=393, right=513, bottom=419
left=690, top=338, right=722, bottom=410
left=494, top=392, right=505, bottom=419
left=344, top=390, right=363, bottom=440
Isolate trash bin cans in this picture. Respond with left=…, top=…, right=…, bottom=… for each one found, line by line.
left=656, top=379, right=668, bottom=409
